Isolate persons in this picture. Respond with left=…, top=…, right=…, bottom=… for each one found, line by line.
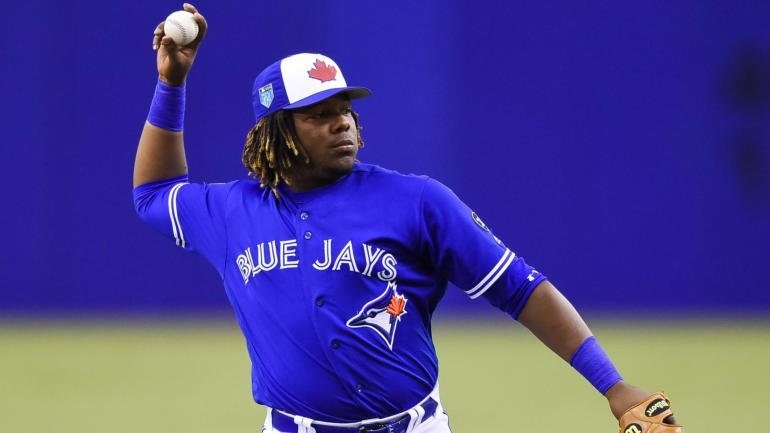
left=132, top=2, right=683, bottom=433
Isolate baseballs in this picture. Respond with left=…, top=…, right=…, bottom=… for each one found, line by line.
left=163, top=9, right=199, bottom=47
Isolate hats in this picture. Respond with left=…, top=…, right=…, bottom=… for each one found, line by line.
left=253, top=54, right=372, bottom=124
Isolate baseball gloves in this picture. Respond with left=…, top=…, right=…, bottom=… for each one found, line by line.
left=619, top=393, right=684, bottom=433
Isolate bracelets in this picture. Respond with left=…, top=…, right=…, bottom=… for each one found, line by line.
left=569, top=336, right=626, bottom=395
left=146, top=77, right=187, bottom=131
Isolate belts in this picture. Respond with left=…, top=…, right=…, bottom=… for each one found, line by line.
left=272, top=399, right=437, bottom=433
